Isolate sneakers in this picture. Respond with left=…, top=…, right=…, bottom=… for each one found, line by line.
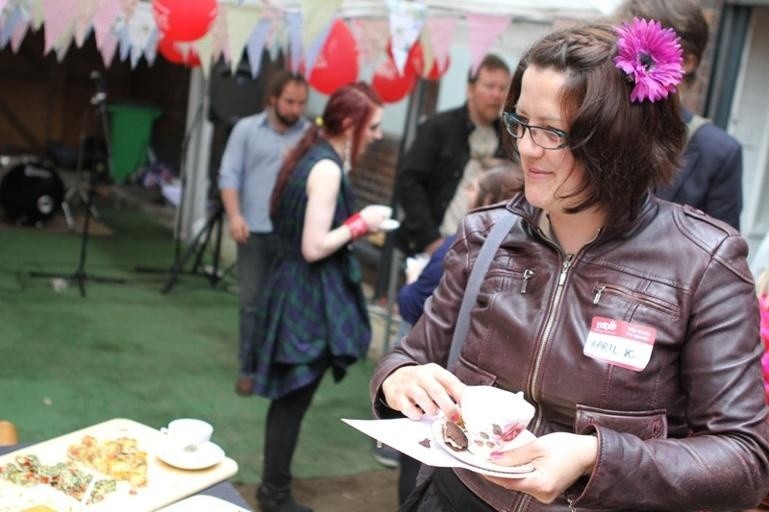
left=254, top=482, right=316, bottom=512
left=234, top=374, right=255, bottom=398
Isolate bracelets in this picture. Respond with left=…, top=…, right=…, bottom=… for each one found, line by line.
left=344, top=213, right=371, bottom=241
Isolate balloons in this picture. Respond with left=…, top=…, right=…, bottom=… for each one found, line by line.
left=152, top=1, right=218, bottom=45
left=154, top=33, right=201, bottom=68
left=286, top=18, right=361, bottom=96
left=368, top=52, right=418, bottom=103
left=388, top=34, right=452, bottom=83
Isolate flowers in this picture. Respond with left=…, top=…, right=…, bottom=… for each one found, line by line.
left=611, top=16, right=686, bottom=103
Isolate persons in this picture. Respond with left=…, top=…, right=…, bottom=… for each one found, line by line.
left=369, top=50, right=521, bottom=472
left=366, top=12, right=767, bottom=511
left=216, top=70, right=316, bottom=395
left=233, top=81, right=403, bottom=512
left=605, top=1, right=747, bottom=232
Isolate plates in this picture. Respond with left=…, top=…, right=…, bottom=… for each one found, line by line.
left=431, top=409, right=540, bottom=473
left=376, top=218, right=400, bottom=231
left=155, top=440, right=226, bottom=470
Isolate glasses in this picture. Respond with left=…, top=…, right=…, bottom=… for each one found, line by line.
left=500, top=110, right=572, bottom=150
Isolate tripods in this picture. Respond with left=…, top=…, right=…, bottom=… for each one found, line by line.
left=159, top=204, right=238, bottom=296
left=29, top=88, right=126, bottom=298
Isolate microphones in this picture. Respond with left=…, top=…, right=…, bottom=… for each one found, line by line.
left=88, top=69, right=104, bottom=92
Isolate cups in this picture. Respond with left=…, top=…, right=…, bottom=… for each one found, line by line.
left=459, top=386, right=535, bottom=450
left=368, top=204, right=393, bottom=225
left=160, top=418, right=214, bottom=456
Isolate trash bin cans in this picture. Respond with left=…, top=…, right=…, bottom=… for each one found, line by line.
left=98, top=105, right=163, bottom=184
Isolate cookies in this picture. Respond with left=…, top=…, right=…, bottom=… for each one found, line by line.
left=441, top=420, right=466, bottom=451
